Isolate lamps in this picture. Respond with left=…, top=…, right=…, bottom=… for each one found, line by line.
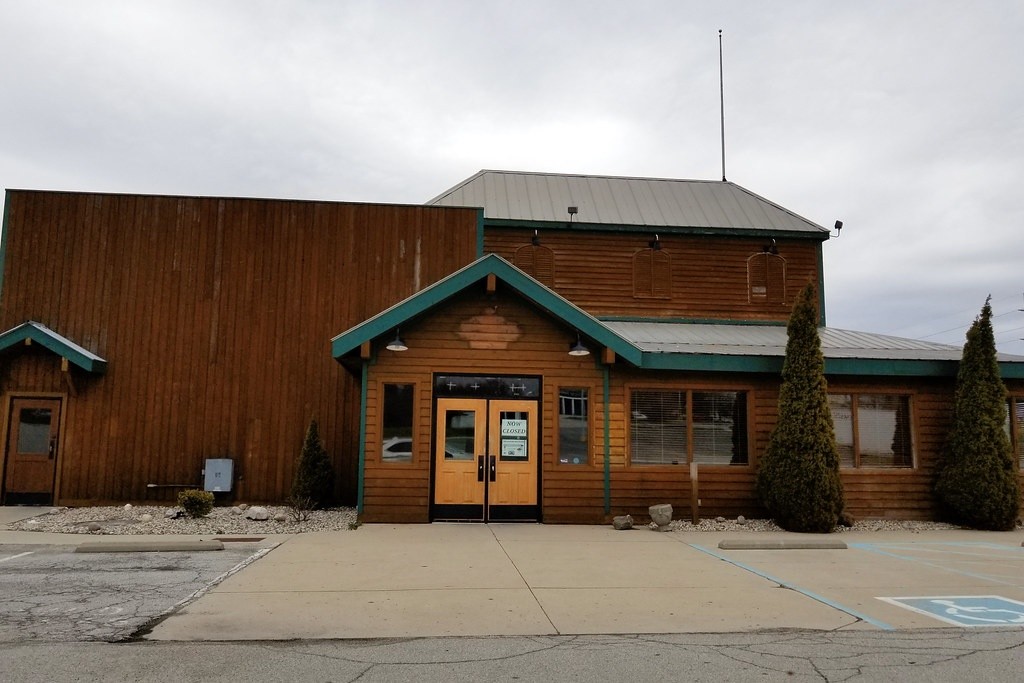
left=648, top=234, right=661, bottom=250
left=481, top=292, right=500, bottom=311
left=532, top=229, right=540, bottom=246
left=386, top=327, right=408, bottom=351
left=568, top=207, right=579, bottom=223
left=568, top=330, right=590, bottom=356
left=829, top=220, right=843, bottom=238
left=764, top=239, right=779, bottom=255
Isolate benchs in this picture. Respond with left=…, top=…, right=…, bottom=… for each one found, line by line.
left=148, top=484, right=198, bottom=509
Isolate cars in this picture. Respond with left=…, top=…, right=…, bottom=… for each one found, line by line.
left=379, top=435, right=474, bottom=460
